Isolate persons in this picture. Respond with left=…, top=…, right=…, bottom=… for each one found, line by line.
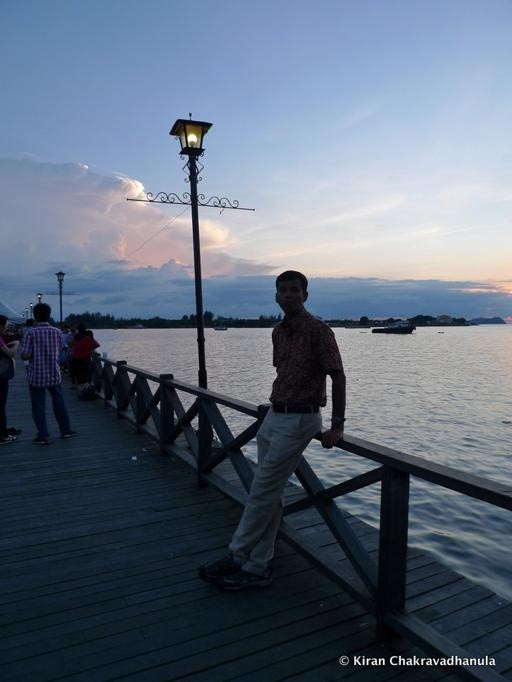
left=4, top=318, right=101, bottom=402
left=0, top=314, right=22, bottom=446
left=18, top=302, right=82, bottom=446
left=196, top=266, right=347, bottom=595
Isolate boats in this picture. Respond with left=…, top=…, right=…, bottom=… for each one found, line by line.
left=127, top=324, right=144, bottom=328
left=372, top=321, right=416, bottom=334
left=214, top=327, right=227, bottom=330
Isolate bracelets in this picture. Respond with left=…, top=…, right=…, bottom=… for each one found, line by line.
left=329, top=415, right=346, bottom=423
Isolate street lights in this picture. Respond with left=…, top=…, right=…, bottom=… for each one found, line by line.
left=54, top=269, right=65, bottom=329
left=29, top=302, right=33, bottom=319
left=125, top=109, right=253, bottom=399
left=25, top=307, right=28, bottom=324
left=22, top=311, right=25, bottom=322
left=36, top=293, right=42, bottom=303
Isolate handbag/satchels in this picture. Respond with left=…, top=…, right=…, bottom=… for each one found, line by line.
left=76, top=383, right=98, bottom=401
left=1, top=356, right=15, bottom=382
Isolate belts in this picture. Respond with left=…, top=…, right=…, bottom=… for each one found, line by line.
left=273, top=402, right=319, bottom=413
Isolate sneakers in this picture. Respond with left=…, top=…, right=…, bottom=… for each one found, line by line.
left=211, top=569, right=272, bottom=589
left=1, top=427, right=78, bottom=446
left=197, top=551, right=242, bottom=583
